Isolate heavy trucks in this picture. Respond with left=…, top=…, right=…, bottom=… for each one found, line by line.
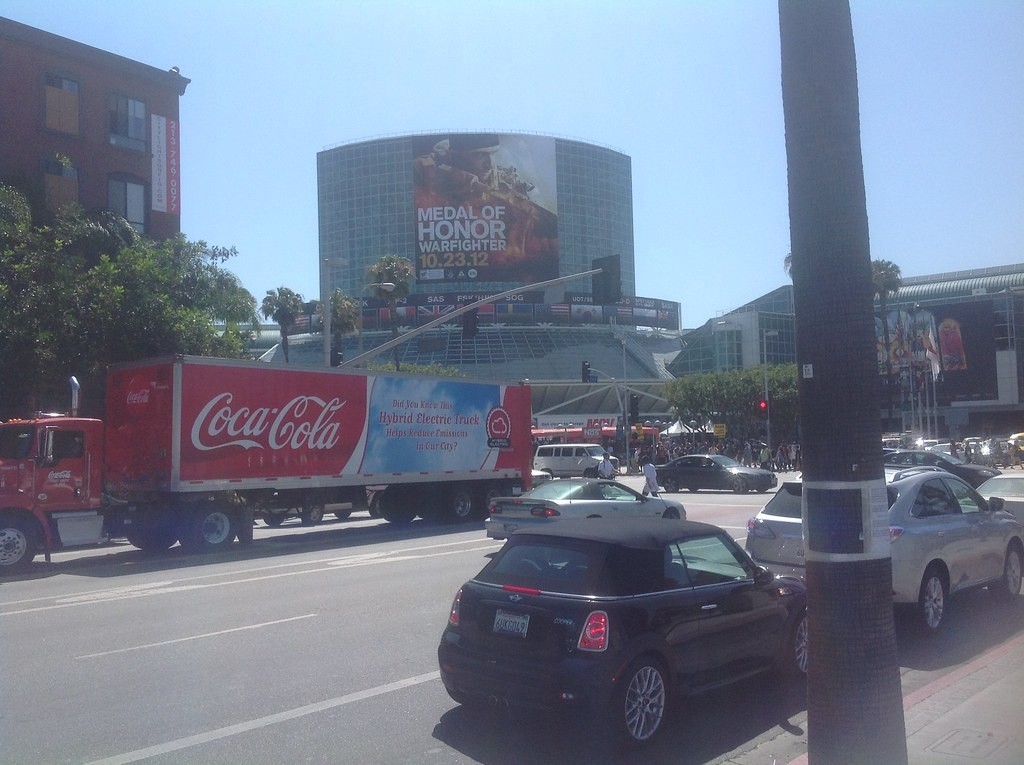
left=1, top=350, right=533, bottom=575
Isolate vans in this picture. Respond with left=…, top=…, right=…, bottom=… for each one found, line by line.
left=534, top=443, right=619, bottom=478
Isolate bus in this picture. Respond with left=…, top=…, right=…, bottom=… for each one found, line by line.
left=531, top=425, right=659, bottom=464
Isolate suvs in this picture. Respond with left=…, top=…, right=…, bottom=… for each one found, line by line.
left=746, top=464, right=1024, bottom=634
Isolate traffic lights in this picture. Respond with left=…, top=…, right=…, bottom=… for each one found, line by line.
left=630, top=394, right=639, bottom=424
left=581, top=360, right=591, bottom=381
left=757, top=400, right=768, bottom=420
left=332, top=348, right=345, bottom=367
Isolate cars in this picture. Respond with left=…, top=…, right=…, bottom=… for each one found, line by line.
left=974, top=472, right=1024, bottom=525
left=255, top=502, right=353, bottom=527
left=485, top=478, right=687, bottom=539
left=436, top=515, right=808, bottom=749
left=884, top=448, right=1002, bottom=490
left=883, top=429, right=1024, bottom=463
left=654, top=453, right=779, bottom=494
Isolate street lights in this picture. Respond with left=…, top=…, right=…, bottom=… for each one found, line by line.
left=718, top=321, right=772, bottom=451
left=353, top=281, right=394, bottom=355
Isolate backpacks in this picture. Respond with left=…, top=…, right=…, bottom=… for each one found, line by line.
left=595, top=460, right=605, bottom=474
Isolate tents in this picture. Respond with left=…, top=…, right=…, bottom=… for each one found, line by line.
left=660, top=418, right=717, bottom=439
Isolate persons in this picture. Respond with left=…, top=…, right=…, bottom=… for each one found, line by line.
left=643, top=455, right=661, bottom=497
left=54, top=430, right=82, bottom=457
left=413, top=132, right=558, bottom=268
left=949, top=437, right=1024, bottom=471
left=882, top=439, right=907, bottom=449
left=597, top=452, right=621, bottom=480
left=633, top=437, right=802, bottom=473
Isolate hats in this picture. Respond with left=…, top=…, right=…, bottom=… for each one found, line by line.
left=761, top=442, right=767, bottom=447
left=448, top=134, right=500, bottom=161
